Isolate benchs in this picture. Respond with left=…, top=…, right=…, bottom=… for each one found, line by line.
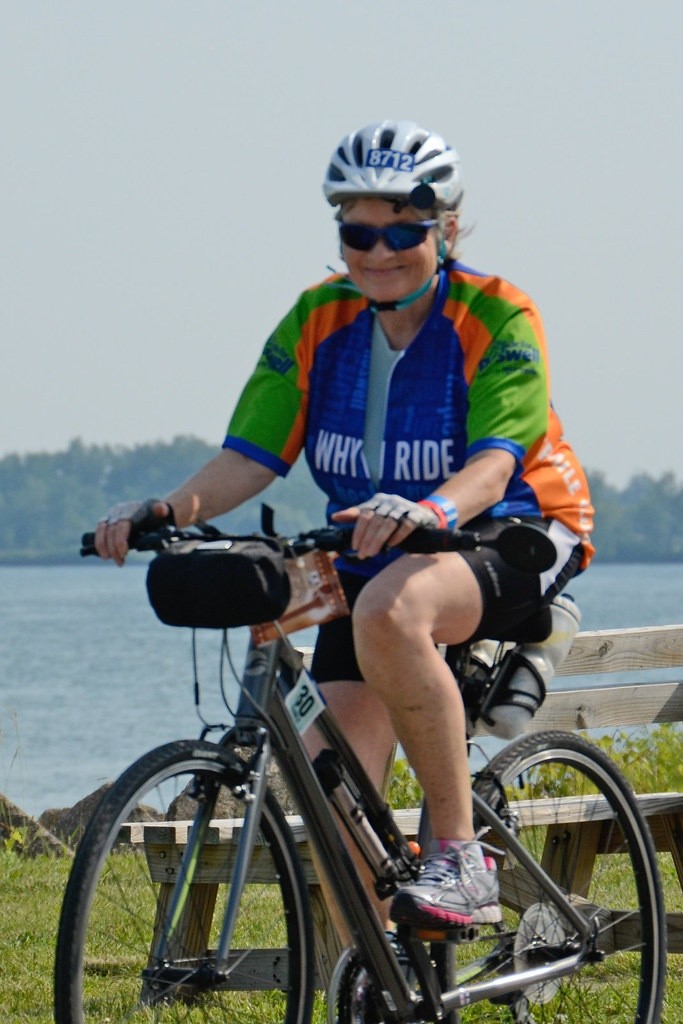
left=110, top=624, right=683, bottom=1004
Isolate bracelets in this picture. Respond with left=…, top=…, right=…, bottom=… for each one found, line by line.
left=414, top=492, right=460, bottom=533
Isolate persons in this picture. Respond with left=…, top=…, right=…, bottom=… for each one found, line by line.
left=92, top=118, right=599, bottom=954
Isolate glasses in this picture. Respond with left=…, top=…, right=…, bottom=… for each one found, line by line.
left=338, top=218, right=440, bottom=251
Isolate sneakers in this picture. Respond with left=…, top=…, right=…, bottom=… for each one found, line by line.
left=390, top=826, right=504, bottom=929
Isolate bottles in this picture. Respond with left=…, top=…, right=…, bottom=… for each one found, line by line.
left=484, top=593, right=582, bottom=740
left=462, top=638, right=517, bottom=738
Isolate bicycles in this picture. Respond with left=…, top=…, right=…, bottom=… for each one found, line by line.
left=53, top=498, right=666, bottom=1024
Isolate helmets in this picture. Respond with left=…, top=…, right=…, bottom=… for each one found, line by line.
left=322, top=117, right=465, bottom=212
